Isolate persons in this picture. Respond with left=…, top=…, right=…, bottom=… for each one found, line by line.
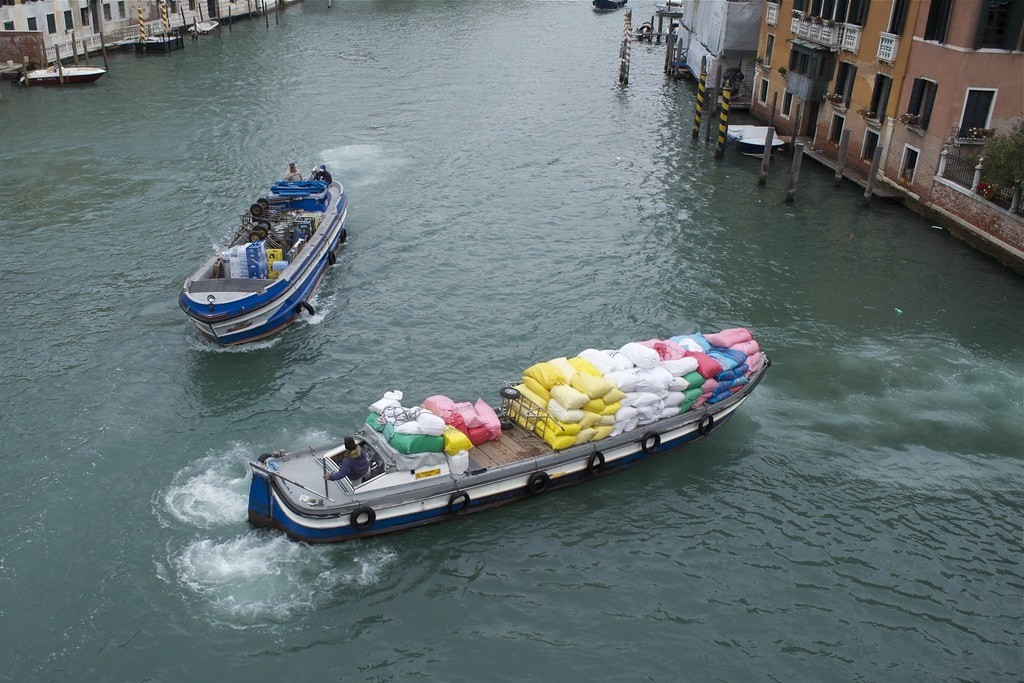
left=314, top=165, right=332, bottom=185
left=282, top=162, right=303, bottom=182
left=323, top=437, right=370, bottom=481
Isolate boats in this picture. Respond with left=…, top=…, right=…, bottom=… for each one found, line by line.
left=592, top=0, right=628, bottom=10
left=727, top=124, right=786, bottom=160
left=246, top=327, right=772, bottom=547
left=20, top=65, right=107, bottom=86
left=188, top=21, right=220, bottom=35
left=654, top=1, right=683, bottom=13
left=179, top=175, right=349, bottom=349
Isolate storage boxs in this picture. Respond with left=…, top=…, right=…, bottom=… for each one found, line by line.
left=293, top=217, right=316, bottom=239
left=221, top=240, right=288, bottom=279
left=267, top=189, right=330, bottom=212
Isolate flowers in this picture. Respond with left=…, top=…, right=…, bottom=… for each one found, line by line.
left=856, top=104, right=875, bottom=118
left=899, top=112, right=921, bottom=125
left=976, top=183, right=995, bottom=200
left=968, top=127, right=978, bottom=139
left=822, top=93, right=842, bottom=103
